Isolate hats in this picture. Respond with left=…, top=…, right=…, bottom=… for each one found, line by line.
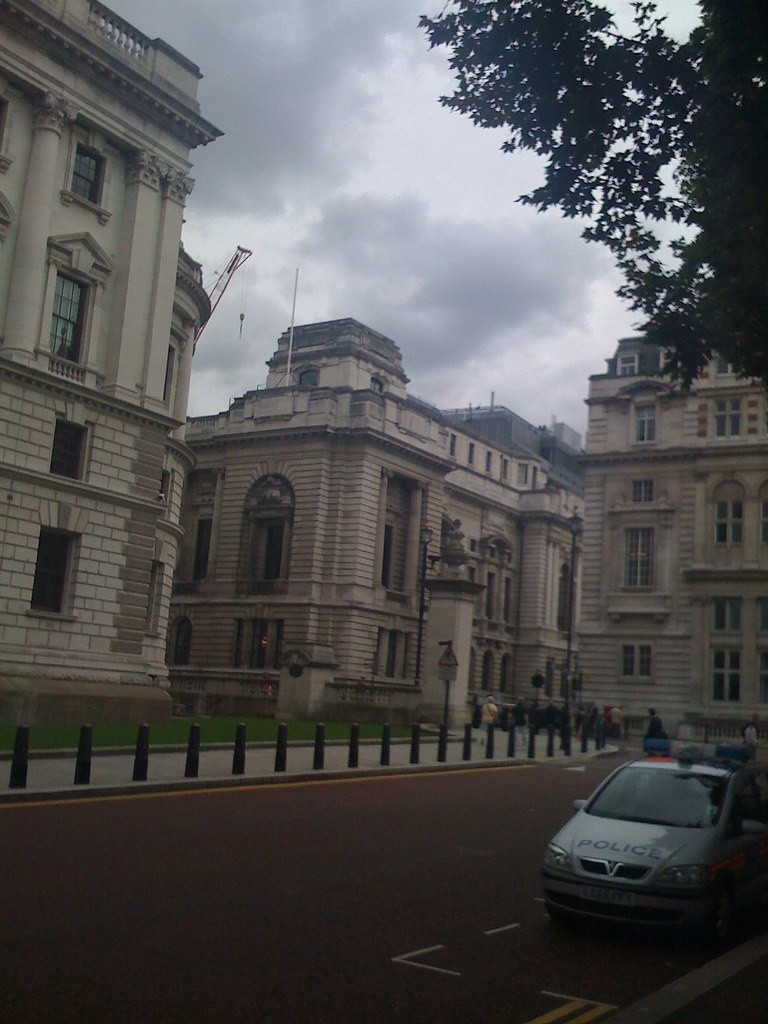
left=648, top=708, right=655, bottom=715
left=488, top=696, right=494, bottom=703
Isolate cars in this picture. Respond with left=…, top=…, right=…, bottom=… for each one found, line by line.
left=538, top=736, right=768, bottom=953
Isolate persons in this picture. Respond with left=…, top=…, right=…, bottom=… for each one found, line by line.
left=740, top=712, right=766, bottom=761
left=643, top=706, right=670, bottom=739
left=466, top=692, right=629, bottom=751
